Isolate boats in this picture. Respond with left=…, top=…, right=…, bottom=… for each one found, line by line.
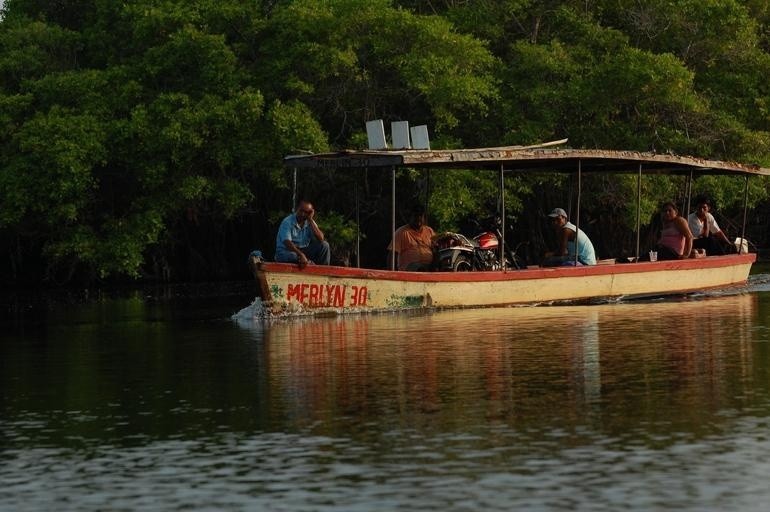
left=250, top=120, right=770, bottom=317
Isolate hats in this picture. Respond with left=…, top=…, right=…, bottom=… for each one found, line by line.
left=548, top=208, right=568, bottom=218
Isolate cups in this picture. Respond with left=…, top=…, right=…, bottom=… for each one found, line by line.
left=649, top=252, right=657, bottom=262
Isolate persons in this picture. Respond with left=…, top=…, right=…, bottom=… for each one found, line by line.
left=539, top=208, right=598, bottom=267
left=684, top=195, right=735, bottom=256
left=386, top=204, right=437, bottom=272
left=274, top=197, right=330, bottom=266
left=616, top=201, right=694, bottom=263
